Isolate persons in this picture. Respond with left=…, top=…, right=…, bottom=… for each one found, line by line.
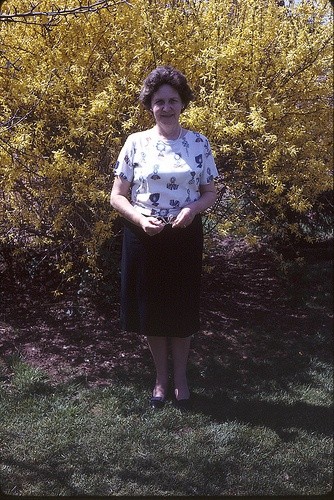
left=111, top=68, right=218, bottom=406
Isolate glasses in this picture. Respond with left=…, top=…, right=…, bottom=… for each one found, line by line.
left=155, top=213, right=178, bottom=226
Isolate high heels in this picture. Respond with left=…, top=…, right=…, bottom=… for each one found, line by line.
left=173, top=379, right=191, bottom=407
left=151, top=375, right=169, bottom=408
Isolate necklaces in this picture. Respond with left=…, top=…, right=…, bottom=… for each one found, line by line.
left=154, top=126, right=182, bottom=146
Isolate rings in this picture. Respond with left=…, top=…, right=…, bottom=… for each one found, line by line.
left=183, top=224, right=186, bottom=228
